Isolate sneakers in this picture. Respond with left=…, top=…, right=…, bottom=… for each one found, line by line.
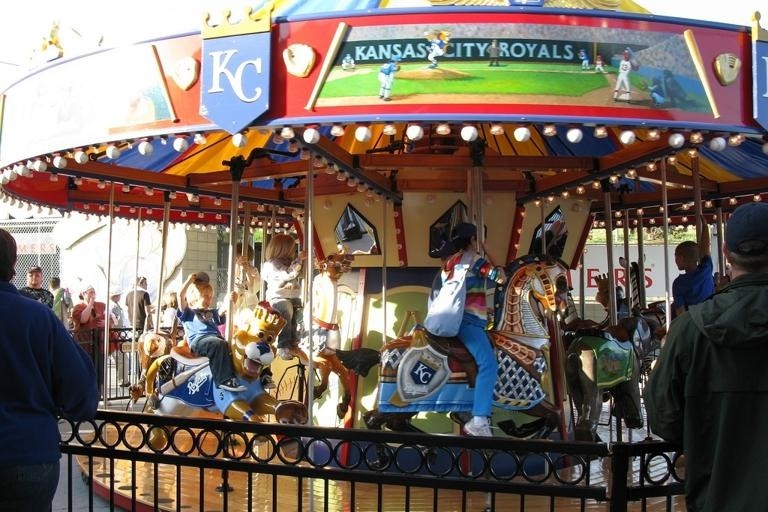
left=262, top=374, right=276, bottom=388
left=276, top=347, right=293, bottom=360
left=462, top=419, right=492, bottom=436
left=219, top=377, right=246, bottom=392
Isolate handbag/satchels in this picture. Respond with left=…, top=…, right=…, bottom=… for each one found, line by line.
left=423, top=271, right=467, bottom=338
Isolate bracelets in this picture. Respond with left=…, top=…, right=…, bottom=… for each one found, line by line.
left=494, top=266, right=501, bottom=270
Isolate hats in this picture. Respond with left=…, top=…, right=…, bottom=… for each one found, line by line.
left=725, top=201, right=767, bottom=256
left=28, top=267, right=42, bottom=273
left=110, top=290, right=121, bottom=298
left=451, top=223, right=475, bottom=240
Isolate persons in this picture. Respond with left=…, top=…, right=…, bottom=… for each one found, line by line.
left=175, top=268, right=247, bottom=393
left=643, top=200, right=768, bottom=512
left=440, top=221, right=508, bottom=433
left=233, top=242, right=260, bottom=311
left=670, top=214, right=714, bottom=314
left=0, top=225, right=95, bottom=512
left=260, top=233, right=313, bottom=353
left=18, top=264, right=180, bottom=403
